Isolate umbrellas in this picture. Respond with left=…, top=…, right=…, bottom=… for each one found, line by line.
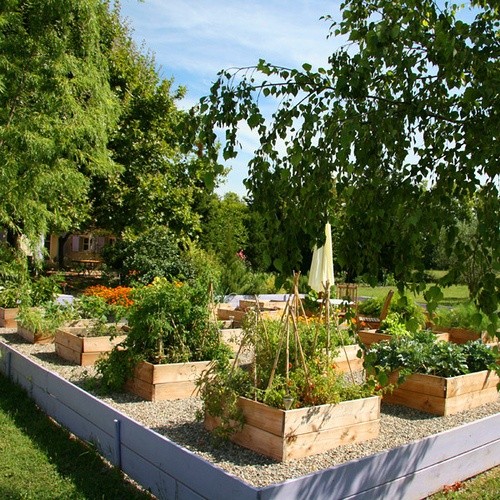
left=308, top=210, right=336, bottom=315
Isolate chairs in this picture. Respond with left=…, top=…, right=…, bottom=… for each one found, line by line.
left=359, top=289, right=394, bottom=329
left=338, top=285, right=359, bottom=314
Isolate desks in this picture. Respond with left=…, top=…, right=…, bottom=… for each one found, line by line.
left=72, top=260, right=100, bottom=270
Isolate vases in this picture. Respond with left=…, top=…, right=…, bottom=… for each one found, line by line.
left=203, top=384, right=381, bottom=462
left=322, top=345, right=364, bottom=371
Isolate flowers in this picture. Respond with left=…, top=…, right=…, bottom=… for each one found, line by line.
left=191, top=310, right=398, bottom=448
left=86, top=276, right=183, bottom=308
left=266, top=308, right=367, bottom=349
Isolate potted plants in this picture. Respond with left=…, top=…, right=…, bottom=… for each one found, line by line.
left=0, top=267, right=500, bottom=461
